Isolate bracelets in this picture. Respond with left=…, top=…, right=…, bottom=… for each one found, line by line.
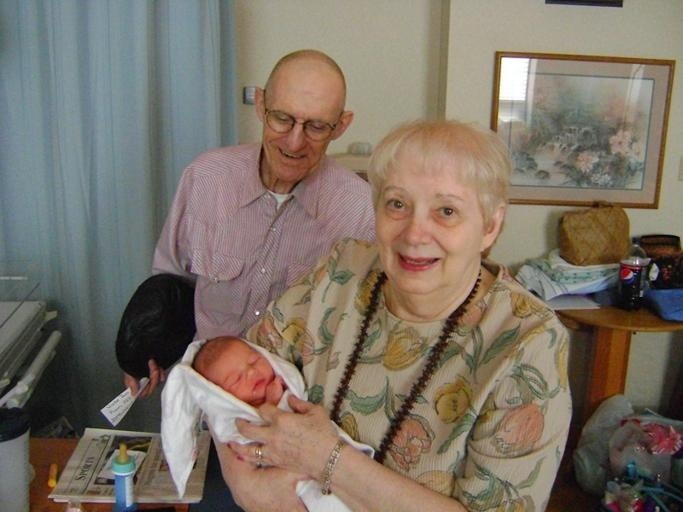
left=317, top=437, right=347, bottom=496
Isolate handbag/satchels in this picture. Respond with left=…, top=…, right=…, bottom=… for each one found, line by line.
left=558, top=204, right=630, bottom=266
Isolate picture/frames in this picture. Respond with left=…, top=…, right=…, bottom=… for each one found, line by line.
left=490, top=50, right=678, bottom=211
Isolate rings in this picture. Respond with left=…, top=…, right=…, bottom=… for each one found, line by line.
left=253, top=459, right=263, bottom=468
left=253, top=444, right=264, bottom=459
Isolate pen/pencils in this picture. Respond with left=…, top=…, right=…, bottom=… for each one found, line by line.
left=48, top=463, right=59, bottom=487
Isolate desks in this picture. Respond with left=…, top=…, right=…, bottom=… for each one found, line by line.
left=538, top=302, right=682, bottom=419
left=21, top=436, right=194, bottom=512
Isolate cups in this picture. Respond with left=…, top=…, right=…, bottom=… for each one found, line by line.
left=0, top=407, right=31, bottom=512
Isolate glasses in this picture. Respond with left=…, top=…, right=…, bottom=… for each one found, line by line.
left=262, top=90, right=340, bottom=141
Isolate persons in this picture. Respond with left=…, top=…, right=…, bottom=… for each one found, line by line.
left=201, top=118, right=575, bottom=511
left=112, top=48, right=374, bottom=401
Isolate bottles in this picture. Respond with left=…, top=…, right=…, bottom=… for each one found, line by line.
left=643, top=472, right=664, bottom=512
left=109, top=442, right=138, bottom=512
left=614, top=238, right=649, bottom=313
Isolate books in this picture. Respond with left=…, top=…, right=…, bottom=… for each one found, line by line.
left=43, top=425, right=211, bottom=506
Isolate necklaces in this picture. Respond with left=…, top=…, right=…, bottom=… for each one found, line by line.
left=329, top=266, right=482, bottom=471
